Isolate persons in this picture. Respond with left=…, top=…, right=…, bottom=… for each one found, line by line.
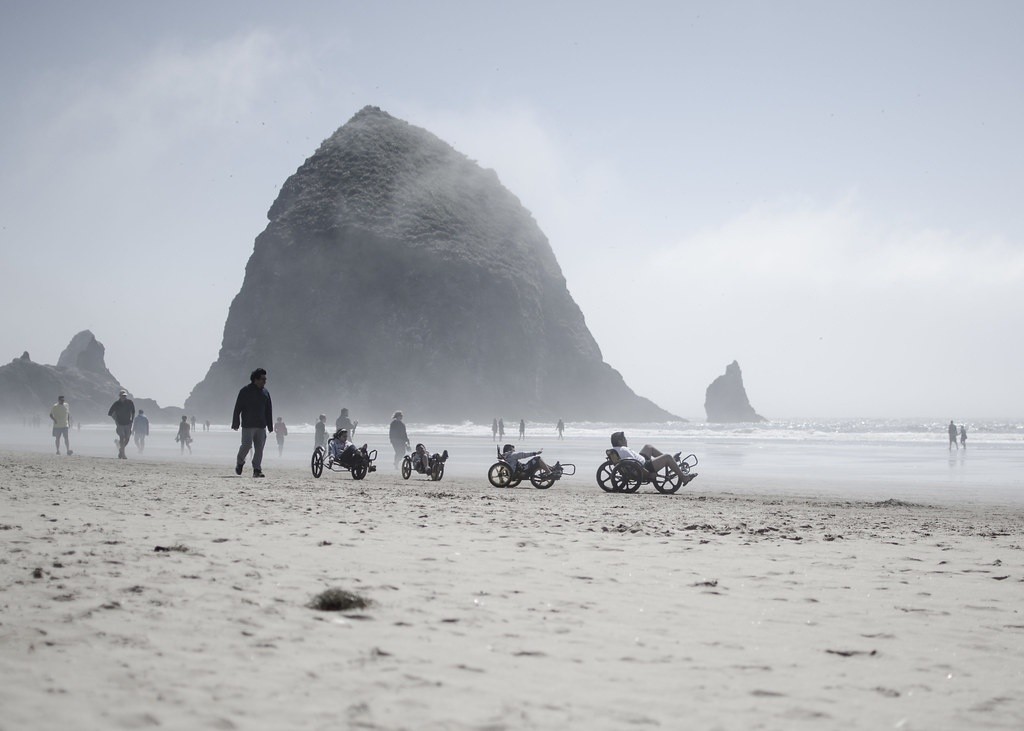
left=503, top=444, right=559, bottom=477
left=176, top=416, right=192, bottom=451
left=413, top=443, right=448, bottom=472
left=333, top=428, right=367, bottom=459
left=108, top=390, right=135, bottom=459
left=49, top=396, right=72, bottom=455
left=21, top=414, right=80, bottom=431
left=274, top=417, right=287, bottom=454
left=133, top=410, right=149, bottom=450
left=611, top=431, right=698, bottom=486
left=519, top=419, right=525, bottom=438
left=498, top=418, right=505, bottom=441
left=389, top=411, right=410, bottom=463
left=203, top=424, right=205, bottom=431
left=231, top=368, right=273, bottom=478
left=191, top=416, right=196, bottom=431
left=492, top=418, right=497, bottom=440
left=556, top=418, right=564, bottom=440
left=947, top=421, right=959, bottom=451
left=336, top=408, right=356, bottom=443
left=957, top=425, right=967, bottom=445
left=314, top=414, right=327, bottom=449
left=206, top=420, right=210, bottom=431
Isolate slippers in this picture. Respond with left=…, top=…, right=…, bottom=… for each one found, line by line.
left=673, top=451, right=681, bottom=460
left=683, top=473, right=698, bottom=486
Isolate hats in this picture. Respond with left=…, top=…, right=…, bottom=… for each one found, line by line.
left=119, top=390, right=127, bottom=395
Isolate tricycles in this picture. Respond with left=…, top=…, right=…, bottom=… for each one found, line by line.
left=311, top=438, right=377, bottom=481
left=596, top=449, right=698, bottom=494
left=402, top=451, right=445, bottom=480
left=486, top=445, right=575, bottom=489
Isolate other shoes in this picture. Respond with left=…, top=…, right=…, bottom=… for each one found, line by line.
left=56, top=452, right=60, bottom=455
left=442, top=450, right=447, bottom=456
left=253, top=470, right=266, bottom=477
left=368, top=465, right=376, bottom=473
left=393, top=461, right=401, bottom=469
left=360, top=444, right=367, bottom=456
left=66, top=450, right=73, bottom=455
left=235, top=465, right=243, bottom=475
left=426, top=467, right=431, bottom=475
left=118, top=454, right=127, bottom=459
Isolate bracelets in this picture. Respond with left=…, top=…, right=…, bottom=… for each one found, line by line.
left=532, top=452, right=536, bottom=455
